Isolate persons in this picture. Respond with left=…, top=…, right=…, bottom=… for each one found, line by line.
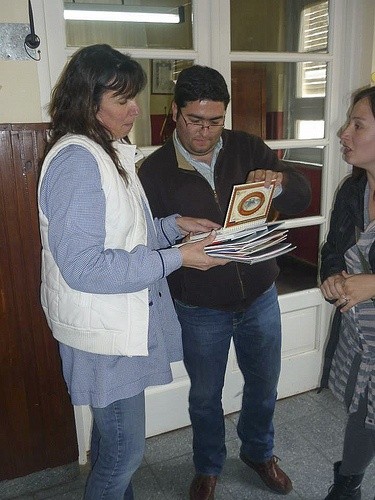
left=316, top=86, right=375, bottom=500
left=138, top=65, right=312, bottom=499
left=36, top=44, right=234, bottom=500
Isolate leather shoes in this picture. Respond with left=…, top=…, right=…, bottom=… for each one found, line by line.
left=190, top=472, right=217, bottom=500
left=240, top=444, right=293, bottom=495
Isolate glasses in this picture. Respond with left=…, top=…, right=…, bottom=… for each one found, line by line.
left=178, top=108, right=226, bottom=133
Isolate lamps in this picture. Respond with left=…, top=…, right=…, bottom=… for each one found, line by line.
left=64, top=2, right=186, bottom=25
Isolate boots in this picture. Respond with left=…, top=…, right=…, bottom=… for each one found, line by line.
left=323, top=460, right=365, bottom=500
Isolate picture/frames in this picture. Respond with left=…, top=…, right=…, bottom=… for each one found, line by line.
left=150, top=58, right=177, bottom=94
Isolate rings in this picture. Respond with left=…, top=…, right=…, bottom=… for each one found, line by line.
left=344, top=296, right=349, bottom=302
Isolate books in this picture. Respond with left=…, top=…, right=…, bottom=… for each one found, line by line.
left=170, top=178, right=298, bottom=265
